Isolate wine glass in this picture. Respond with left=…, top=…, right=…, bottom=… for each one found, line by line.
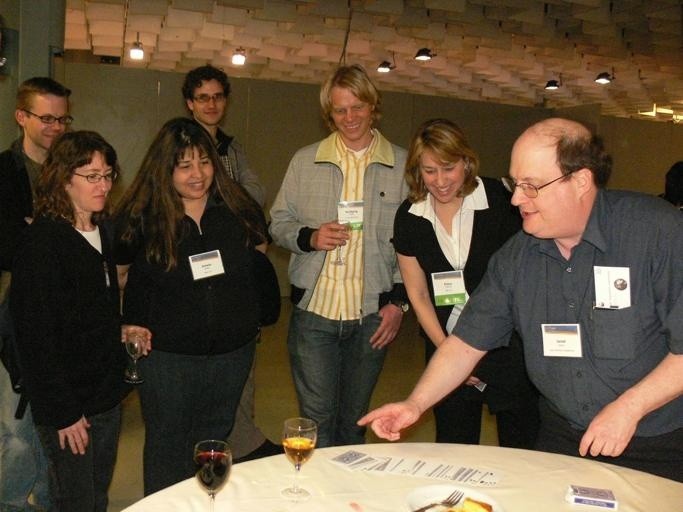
left=282, top=418, right=319, bottom=502
left=191, top=439, right=233, bottom=509
left=121, top=330, right=147, bottom=386
left=330, top=221, right=352, bottom=267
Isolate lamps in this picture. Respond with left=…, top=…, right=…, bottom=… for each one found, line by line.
left=545, top=73, right=563, bottom=89
left=378, top=52, right=397, bottom=72
left=232, top=46, right=245, bottom=65
left=130, top=32, right=143, bottom=59
left=414, top=48, right=438, bottom=60
left=594, top=67, right=616, bottom=85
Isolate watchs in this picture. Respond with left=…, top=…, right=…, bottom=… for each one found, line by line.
left=384, top=295, right=410, bottom=313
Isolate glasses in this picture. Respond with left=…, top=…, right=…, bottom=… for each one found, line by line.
left=73, top=171, right=118, bottom=184
left=22, top=108, right=75, bottom=126
left=500, top=168, right=582, bottom=199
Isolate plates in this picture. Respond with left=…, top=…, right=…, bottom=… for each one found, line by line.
left=403, top=485, right=498, bottom=512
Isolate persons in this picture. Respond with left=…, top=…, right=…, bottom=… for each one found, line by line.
left=661, top=159, right=683, bottom=204
left=391, top=118, right=529, bottom=449
left=269, top=62, right=414, bottom=454
left=1, top=77, right=76, bottom=512
left=110, top=117, right=270, bottom=497
left=180, top=63, right=291, bottom=464
left=357, top=118, right=682, bottom=483
left=19, top=132, right=153, bottom=512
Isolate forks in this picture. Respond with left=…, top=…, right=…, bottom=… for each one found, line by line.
left=409, top=484, right=465, bottom=512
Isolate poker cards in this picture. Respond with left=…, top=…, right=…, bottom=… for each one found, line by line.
left=331, top=450, right=498, bottom=489
